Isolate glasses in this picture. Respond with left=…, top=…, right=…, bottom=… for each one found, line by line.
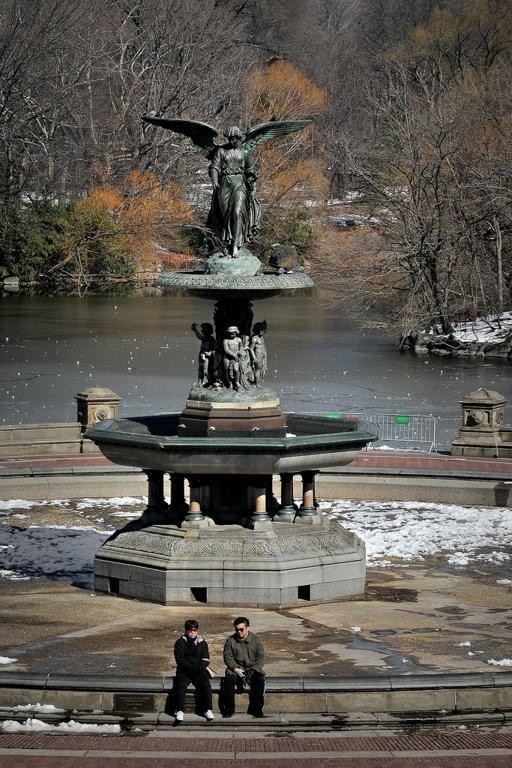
left=235, top=627, right=246, bottom=632
left=187, top=629, right=198, bottom=633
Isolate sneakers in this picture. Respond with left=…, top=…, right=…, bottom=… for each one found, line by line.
left=174, top=708, right=265, bottom=722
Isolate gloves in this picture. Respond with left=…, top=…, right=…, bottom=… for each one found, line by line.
left=242, top=669, right=254, bottom=682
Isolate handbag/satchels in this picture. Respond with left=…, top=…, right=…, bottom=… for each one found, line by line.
left=202, top=657, right=218, bottom=679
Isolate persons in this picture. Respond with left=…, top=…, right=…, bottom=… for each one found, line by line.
left=220, top=617, right=267, bottom=719
left=191, top=316, right=269, bottom=391
left=139, top=115, right=317, bottom=259
left=170, top=620, right=216, bottom=723
